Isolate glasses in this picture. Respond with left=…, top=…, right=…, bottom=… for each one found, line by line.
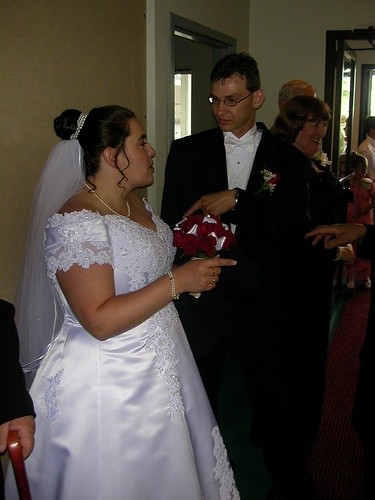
left=208, top=87, right=260, bottom=107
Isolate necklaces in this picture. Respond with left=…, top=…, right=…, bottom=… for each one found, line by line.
left=84, top=183, right=131, bottom=219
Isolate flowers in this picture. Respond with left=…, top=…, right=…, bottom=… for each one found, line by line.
left=174, top=213, right=238, bottom=299
left=253, top=169, right=280, bottom=196
left=312, top=149, right=333, bottom=169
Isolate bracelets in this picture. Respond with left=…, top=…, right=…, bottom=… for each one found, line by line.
left=166, top=271, right=180, bottom=301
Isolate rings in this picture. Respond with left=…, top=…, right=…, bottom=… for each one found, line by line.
left=212, top=268, right=216, bottom=276
left=212, top=277, right=216, bottom=282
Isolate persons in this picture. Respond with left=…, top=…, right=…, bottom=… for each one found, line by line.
left=5, top=107, right=240, bottom=500
left=1, top=299, right=35, bottom=500
left=343, top=151, right=375, bottom=292
left=356, top=116, right=375, bottom=264
left=303, top=223, right=375, bottom=500
left=161, top=52, right=353, bottom=500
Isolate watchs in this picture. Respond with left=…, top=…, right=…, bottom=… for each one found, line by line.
left=235, top=187, right=239, bottom=203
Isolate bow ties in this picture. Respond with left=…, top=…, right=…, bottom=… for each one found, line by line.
left=223, top=134, right=254, bottom=153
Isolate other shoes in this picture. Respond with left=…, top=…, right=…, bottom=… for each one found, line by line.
left=345, top=275, right=354, bottom=288
left=364, top=275, right=371, bottom=289
